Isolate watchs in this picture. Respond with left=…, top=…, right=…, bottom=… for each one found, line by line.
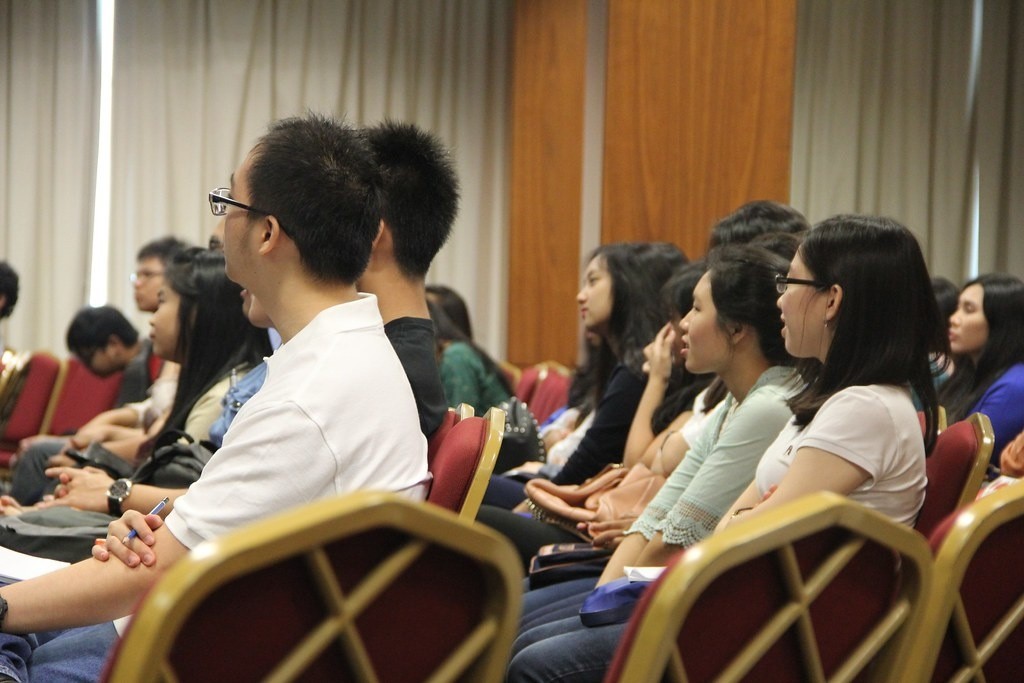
left=107, top=477, right=134, bottom=517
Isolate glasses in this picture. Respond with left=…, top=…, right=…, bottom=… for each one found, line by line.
left=208, top=188, right=297, bottom=240
left=77, top=345, right=99, bottom=366
left=774, top=273, right=835, bottom=294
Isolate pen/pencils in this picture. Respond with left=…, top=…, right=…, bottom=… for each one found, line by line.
left=120, top=496, right=171, bottom=546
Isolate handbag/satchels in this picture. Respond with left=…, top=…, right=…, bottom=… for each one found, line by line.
left=492, top=396, right=547, bottom=476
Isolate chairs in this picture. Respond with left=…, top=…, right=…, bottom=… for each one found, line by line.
left=99, top=490, right=522, bottom=682
left=913, top=405, right=994, bottom=534
left=1, top=349, right=123, bottom=479
left=426, top=361, right=578, bottom=522
left=928, top=477, right=1023, bottom=683
left=602, top=495, right=934, bottom=683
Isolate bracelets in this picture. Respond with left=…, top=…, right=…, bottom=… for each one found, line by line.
left=0, top=595, right=8, bottom=622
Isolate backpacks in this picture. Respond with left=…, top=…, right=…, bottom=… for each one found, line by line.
left=127, top=429, right=220, bottom=490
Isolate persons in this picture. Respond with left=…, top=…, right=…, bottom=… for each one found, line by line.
left=506, top=214, right=952, bottom=683
left=423, top=284, right=517, bottom=418
left=945, top=273, right=1024, bottom=466
left=910, top=275, right=961, bottom=410
left=1, top=120, right=459, bottom=587
left=474, top=199, right=814, bottom=640
left=0, top=117, right=429, bottom=683
